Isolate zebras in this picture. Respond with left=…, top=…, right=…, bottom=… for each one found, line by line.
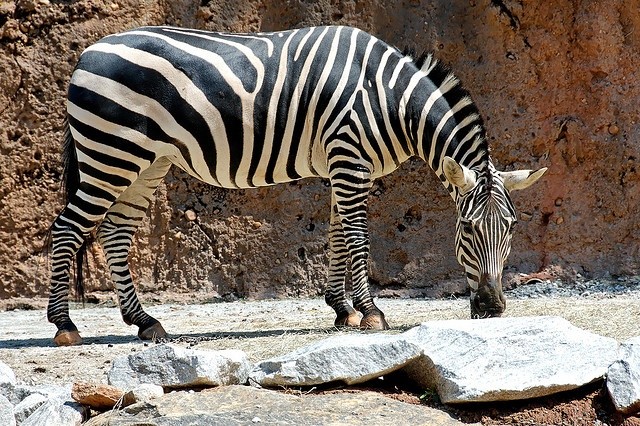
left=37, top=23, right=549, bottom=346
